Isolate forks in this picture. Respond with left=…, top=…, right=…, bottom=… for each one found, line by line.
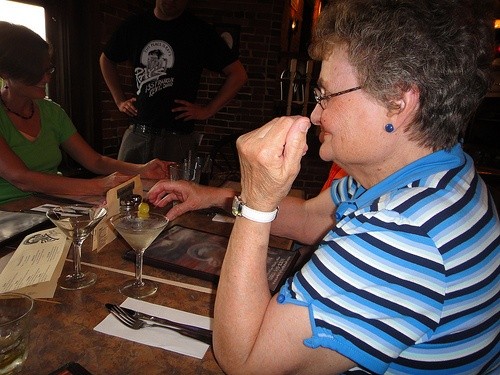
left=109, top=304, right=212, bottom=345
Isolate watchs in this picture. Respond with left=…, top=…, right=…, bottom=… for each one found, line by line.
left=232, top=194, right=279, bottom=223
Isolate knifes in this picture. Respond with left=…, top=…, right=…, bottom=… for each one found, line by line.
left=105, top=303, right=212, bottom=337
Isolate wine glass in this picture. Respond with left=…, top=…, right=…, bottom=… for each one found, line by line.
left=109, top=211, right=169, bottom=297
left=45, top=205, right=107, bottom=290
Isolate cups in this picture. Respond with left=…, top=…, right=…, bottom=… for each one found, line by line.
left=170, top=164, right=200, bottom=184
left=0, top=292, right=33, bottom=374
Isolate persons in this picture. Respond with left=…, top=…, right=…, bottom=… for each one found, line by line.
left=99, top=0, right=248, bottom=164
left=0, top=21, right=176, bottom=204
left=146, top=0, right=500, bottom=375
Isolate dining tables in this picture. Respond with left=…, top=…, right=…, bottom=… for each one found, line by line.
left=1, top=185, right=309, bottom=375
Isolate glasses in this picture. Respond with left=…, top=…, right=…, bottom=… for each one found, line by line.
left=310, top=82, right=366, bottom=102
left=39, top=63, right=58, bottom=76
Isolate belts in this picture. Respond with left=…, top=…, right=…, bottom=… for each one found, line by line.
left=126, top=119, right=188, bottom=140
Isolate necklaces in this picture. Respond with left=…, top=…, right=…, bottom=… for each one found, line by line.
left=0, top=95, right=35, bottom=120
